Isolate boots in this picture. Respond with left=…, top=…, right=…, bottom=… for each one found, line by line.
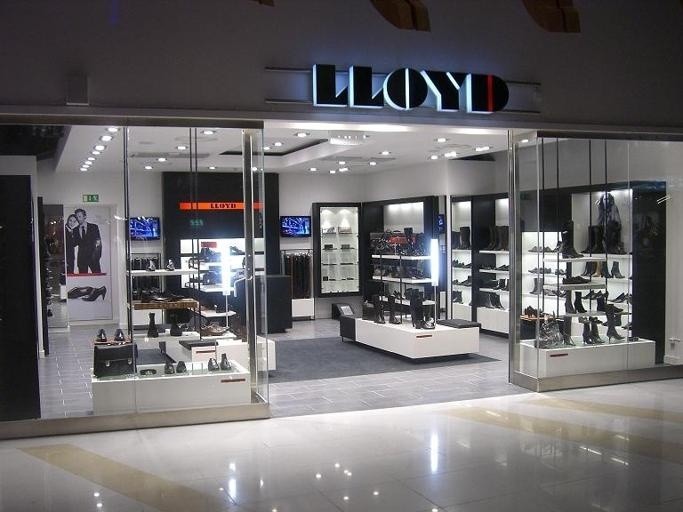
left=563, top=289, right=633, bottom=345
left=362, top=287, right=436, bottom=330
left=451, top=225, right=510, bottom=311
left=404, top=226, right=417, bottom=256
left=527, top=220, right=633, bottom=304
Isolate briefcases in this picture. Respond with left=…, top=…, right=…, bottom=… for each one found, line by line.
left=93, top=342, right=139, bottom=377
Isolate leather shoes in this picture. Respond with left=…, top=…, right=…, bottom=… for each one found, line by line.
left=133, top=259, right=185, bottom=338
left=97, top=328, right=125, bottom=342
left=207, top=353, right=232, bottom=371
left=164, top=360, right=187, bottom=375
left=372, top=265, right=432, bottom=280
left=200, top=323, right=231, bottom=337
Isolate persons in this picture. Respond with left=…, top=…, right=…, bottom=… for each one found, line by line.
left=64, top=214, right=78, bottom=274
left=65, top=208, right=102, bottom=274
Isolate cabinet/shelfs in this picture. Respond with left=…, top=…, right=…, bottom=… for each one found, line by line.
left=126, top=267, right=199, bottom=344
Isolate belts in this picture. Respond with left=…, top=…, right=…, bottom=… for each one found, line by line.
left=283, top=253, right=311, bottom=298
left=628, top=336, right=639, bottom=342
left=140, top=369, right=157, bottom=376
left=132, top=257, right=160, bottom=301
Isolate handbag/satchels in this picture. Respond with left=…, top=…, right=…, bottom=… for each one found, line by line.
left=322, top=227, right=337, bottom=234
left=337, top=226, right=352, bottom=234
left=533, top=317, right=564, bottom=349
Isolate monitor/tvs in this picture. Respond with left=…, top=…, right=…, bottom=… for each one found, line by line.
left=129, top=217, right=161, bottom=241
left=280, top=216, right=312, bottom=238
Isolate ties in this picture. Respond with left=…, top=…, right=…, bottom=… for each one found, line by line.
left=82, top=225, right=85, bottom=239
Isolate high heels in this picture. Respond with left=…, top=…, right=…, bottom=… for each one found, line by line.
left=68, top=286, right=107, bottom=302
left=524, top=305, right=549, bottom=318
